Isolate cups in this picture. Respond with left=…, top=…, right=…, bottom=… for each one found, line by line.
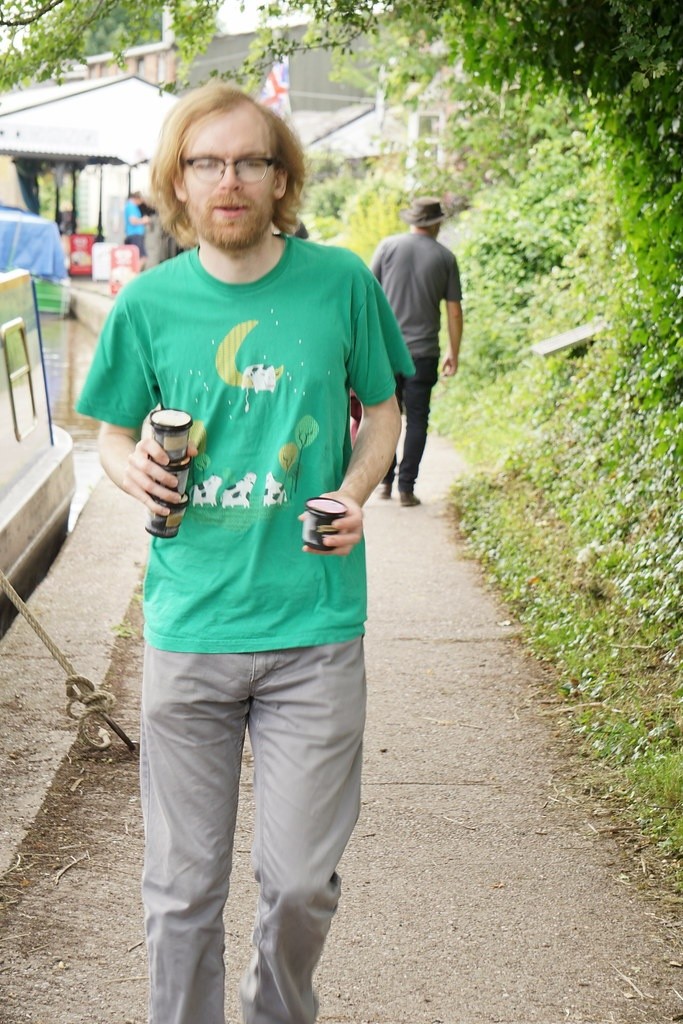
left=153, top=459, right=190, bottom=496
left=301, top=497, right=349, bottom=551
left=150, top=409, right=193, bottom=462
left=144, top=491, right=192, bottom=538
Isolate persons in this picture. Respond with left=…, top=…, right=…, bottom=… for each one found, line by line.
left=124, top=190, right=153, bottom=272
left=75, top=75, right=415, bottom=1024
left=369, top=195, right=462, bottom=508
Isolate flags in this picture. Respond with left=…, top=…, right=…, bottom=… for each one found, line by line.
left=253, top=51, right=294, bottom=126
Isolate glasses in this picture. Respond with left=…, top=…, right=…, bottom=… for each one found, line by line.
left=179, top=156, right=274, bottom=184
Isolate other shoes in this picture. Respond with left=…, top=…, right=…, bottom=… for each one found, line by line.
left=399, top=491, right=421, bottom=506
left=373, top=484, right=392, bottom=499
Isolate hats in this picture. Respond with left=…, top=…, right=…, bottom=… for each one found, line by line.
left=398, top=197, right=447, bottom=227
